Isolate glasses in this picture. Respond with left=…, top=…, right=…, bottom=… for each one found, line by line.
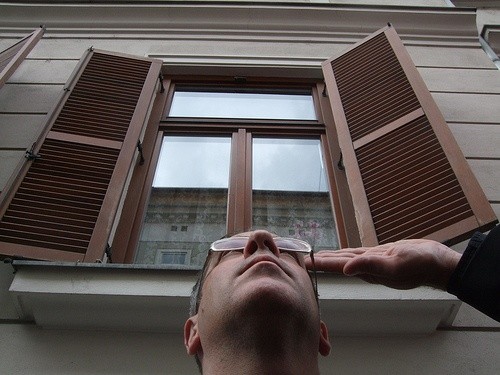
left=195, top=236, right=318, bottom=315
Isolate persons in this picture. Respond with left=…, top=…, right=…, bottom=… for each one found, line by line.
left=184, top=222, right=500, bottom=375
